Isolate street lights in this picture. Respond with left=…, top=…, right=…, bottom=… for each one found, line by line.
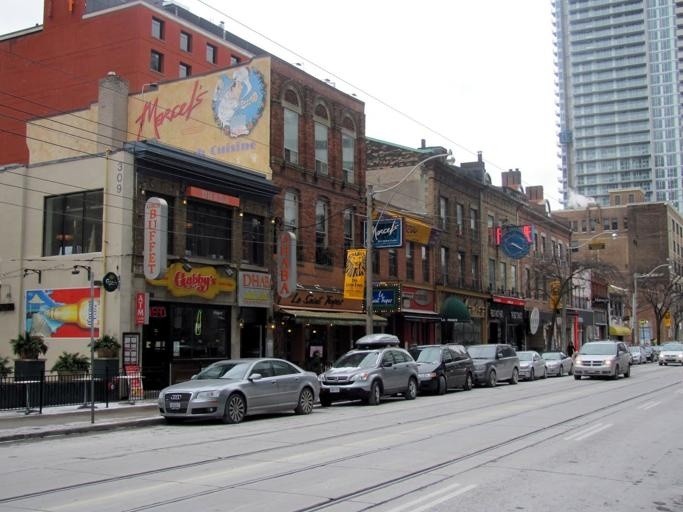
left=364, top=150, right=456, bottom=333
left=567, top=230, right=618, bottom=350
left=631, top=261, right=673, bottom=346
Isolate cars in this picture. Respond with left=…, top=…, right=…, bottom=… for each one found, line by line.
left=629, top=340, right=683, bottom=367
left=515, top=349, right=548, bottom=381
left=467, top=344, right=520, bottom=388
left=572, top=340, right=633, bottom=381
left=158, top=357, right=321, bottom=425
left=542, top=351, right=573, bottom=378
left=408, top=342, right=477, bottom=396
left=318, top=334, right=420, bottom=405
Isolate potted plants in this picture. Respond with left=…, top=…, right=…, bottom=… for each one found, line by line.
left=88, top=333, right=121, bottom=358
left=11, top=330, right=48, bottom=359
left=50, top=351, right=90, bottom=381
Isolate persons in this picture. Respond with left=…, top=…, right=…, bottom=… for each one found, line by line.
left=650, top=338, right=654, bottom=345
left=566, top=341, right=575, bottom=356
left=653, top=336, right=657, bottom=345
